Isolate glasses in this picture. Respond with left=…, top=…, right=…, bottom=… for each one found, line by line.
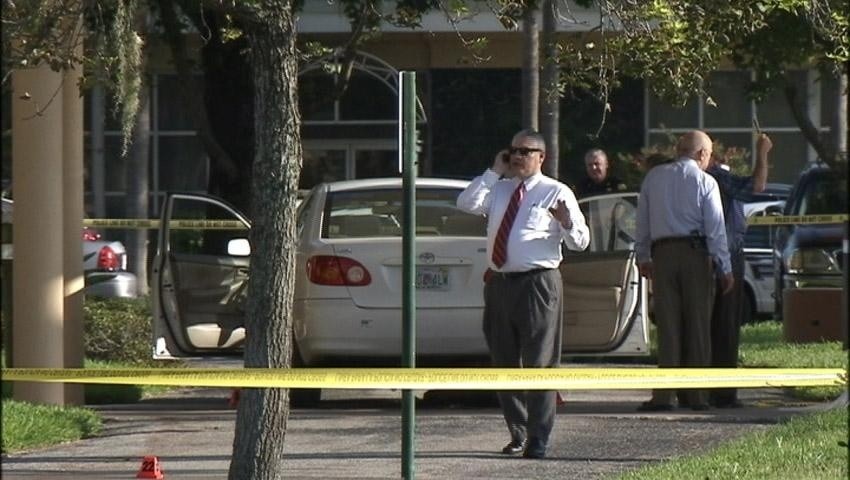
left=509, top=146, right=541, bottom=155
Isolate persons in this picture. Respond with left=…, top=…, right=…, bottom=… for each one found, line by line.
left=569, top=148, right=638, bottom=254
left=703, top=127, right=773, bottom=409
left=631, top=129, right=734, bottom=411
left=457, top=128, right=593, bottom=458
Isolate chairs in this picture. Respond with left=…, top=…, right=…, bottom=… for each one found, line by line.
left=336, top=204, right=445, bottom=235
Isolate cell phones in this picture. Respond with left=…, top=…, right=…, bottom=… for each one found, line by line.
left=503, top=154, right=510, bottom=163
left=751, top=118, right=760, bottom=134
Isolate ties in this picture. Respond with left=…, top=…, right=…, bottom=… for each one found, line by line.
left=491, top=182, right=525, bottom=269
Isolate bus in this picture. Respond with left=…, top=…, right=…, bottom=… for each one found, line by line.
left=0, top=198, right=127, bottom=272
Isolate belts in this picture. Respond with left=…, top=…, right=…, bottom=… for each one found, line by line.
left=493, top=268, right=550, bottom=279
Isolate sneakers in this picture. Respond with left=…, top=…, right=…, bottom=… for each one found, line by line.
left=638, top=398, right=744, bottom=412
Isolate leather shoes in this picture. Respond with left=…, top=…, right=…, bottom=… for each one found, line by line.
left=503, top=440, right=545, bottom=459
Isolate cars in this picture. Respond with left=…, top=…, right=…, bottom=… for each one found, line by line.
left=743, top=166, right=850, bottom=342
left=150, top=175, right=652, bottom=408
